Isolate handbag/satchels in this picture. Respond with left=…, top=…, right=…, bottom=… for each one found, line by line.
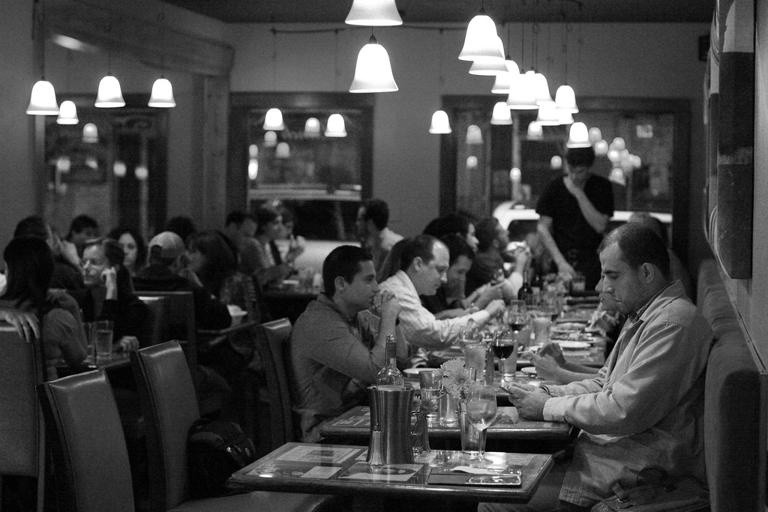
left=186, top=410, right=257, bottom=499
left=591, top=465, right=711, bottom=511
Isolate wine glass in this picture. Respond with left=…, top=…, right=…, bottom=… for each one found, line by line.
left=460, top=328, right=479, bottom=353
left=490, top=269, right=504, bottom=288
left=508, top=300, right=527, bottom=355
left=492, top=331, right=514, bottom=386
left=467, top=388, right=498, bottom=467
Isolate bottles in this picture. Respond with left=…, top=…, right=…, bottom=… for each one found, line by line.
left=518, top=270, right=533, bottom=312
left=378, top=334, right=404, bottom=390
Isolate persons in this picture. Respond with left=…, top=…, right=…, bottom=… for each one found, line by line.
left=291, top=200, right=541, bottom=439
left=477, top=222, right=715, bottom=509
left=626, top=213, right=697, bottom=304
left=529, top=266, right=628, bottom=384
left=1, top=206, right=304, bottom=381
left=536, top=146, right=614, bottom=295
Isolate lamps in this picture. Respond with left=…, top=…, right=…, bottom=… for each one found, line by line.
left=25, top=0, right=177, bottom=145
left=344, top=1, right=403, bottom=27
left=246, top=28, right=348, bottom=180
left=428, top=0, right=642, bottom=186
left=349, top=27, right=399, bottom=94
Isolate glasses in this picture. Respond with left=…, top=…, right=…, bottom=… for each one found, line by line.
left=74, top=227, right=93, bottom=236
left=119, top=244, right=137, bottom=248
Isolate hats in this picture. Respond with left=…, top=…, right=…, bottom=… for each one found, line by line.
left=147, top=231, right=187, bottom=262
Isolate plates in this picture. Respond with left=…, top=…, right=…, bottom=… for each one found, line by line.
left=520, top=367, right=536, bottom=375
left=552, top=340, right=591, bottom=350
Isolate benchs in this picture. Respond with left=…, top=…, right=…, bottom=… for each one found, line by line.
left=695, top=258, right=768, bottom=512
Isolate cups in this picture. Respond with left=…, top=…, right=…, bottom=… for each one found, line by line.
left=421, top=369, right=444, bottom=425
left=533, top=316, right=552, bottom=348
left=93, top=321, right=114, bottom=361
left=500, top=330, right=518, bottom=379
left=464, top=345, right=488, bottom=384
